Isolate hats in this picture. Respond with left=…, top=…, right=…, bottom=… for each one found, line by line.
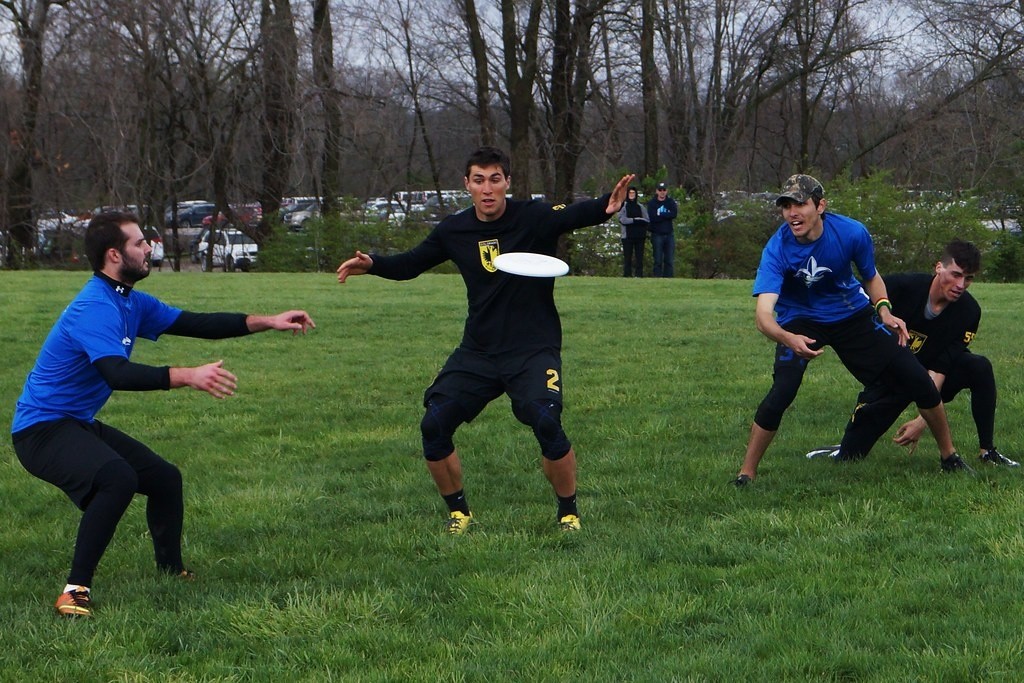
left=775, top=173, right=826, bottom=205
left=656, top=182, right=668, bottom=189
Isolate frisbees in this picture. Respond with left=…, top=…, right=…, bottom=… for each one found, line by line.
left=490, top=249, right=573, bottom=279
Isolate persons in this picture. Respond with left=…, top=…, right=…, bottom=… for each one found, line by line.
left=647, top=182, right=679, bottom=278
left=10, top=207, right=318, bottom=620
left=726, top=173, right=979, bottom=491
left=338, top=147, right=636, bottom=542
left=617, top=186, right=650, bottom=279
left=804, top=237, right=1024, bottom=469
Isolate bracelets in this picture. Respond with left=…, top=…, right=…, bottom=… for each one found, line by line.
left=872, top=297, right=892, bottom=313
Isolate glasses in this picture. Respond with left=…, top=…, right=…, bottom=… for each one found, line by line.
left=658, top=188, right=667, bottom=191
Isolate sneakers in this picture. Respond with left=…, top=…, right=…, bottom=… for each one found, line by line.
left=978, top=447, right=1021, bottom=470
left=55, top=585, right=94, bottom=619
left=941, top=453, right=979, bottom=477
left=557, top=514, right=581, bottom=533
left=176, top=569, right=197, bottom=581
left=728, top=474, right=755, bottom=490
left=446, top=510, right=474, bottom=542
left=805, top=445, right=841, bottom=461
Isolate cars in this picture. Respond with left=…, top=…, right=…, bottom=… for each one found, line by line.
left=36, top=211, right=80, bottom=232
left=234, top=197, right=362, bottom=222
left=202, top=205, right=263, bottom=228
left=952, top=197, right=1022, bottom=246
left=77, top=219, right=92, bottom=229
left=199, top=230, right=259, bottom=273
left=715, top=188, right=1021, bottom=222
left=189, top=220, right=258, bottom=264
left=164, top=200, right=209, bottom=216
left=165, top=204, right=216, bottom=228
left=365, top=191, right=690, bottom=261
left=91, top=204, right=148, bottom=216
left=283, top=200, right=362, bottom=232
left=36, top=233, right=52, bottom=256
left=144, top=225, right=164, bottom=267
